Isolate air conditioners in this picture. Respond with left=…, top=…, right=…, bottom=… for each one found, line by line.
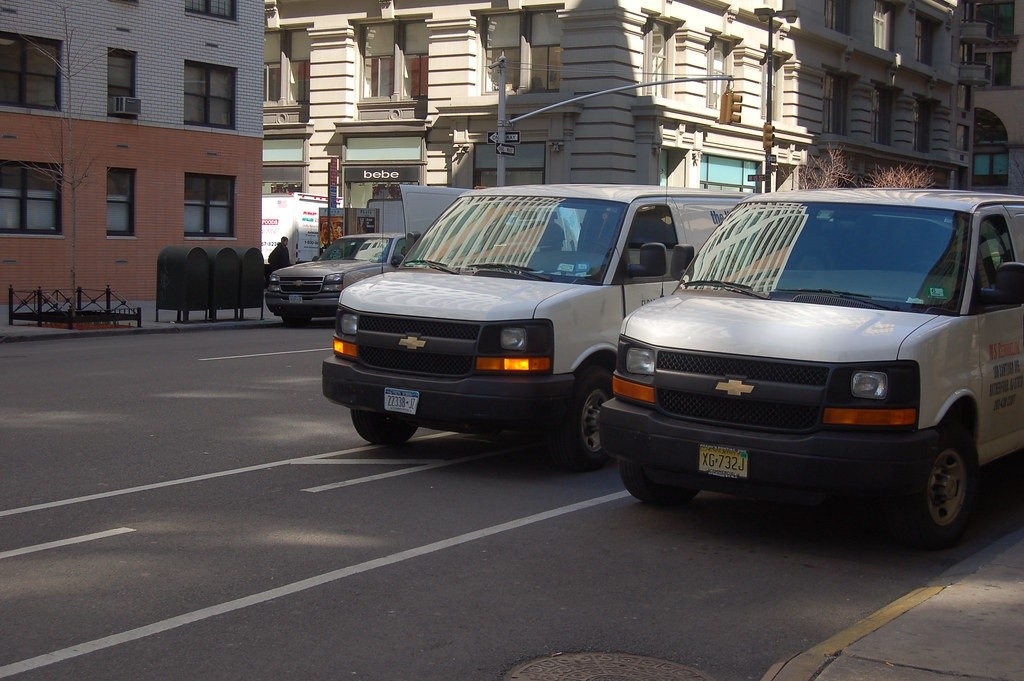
left=112, top=96, right=142, bottom=115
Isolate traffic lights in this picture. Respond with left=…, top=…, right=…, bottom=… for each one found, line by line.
left=763, top=122, right=775, bottom=149
left=725, top=93, right=743, bottom=123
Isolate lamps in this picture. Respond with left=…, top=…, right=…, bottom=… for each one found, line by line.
left=270, top=183, right=277, bottom=189
left=358, top=183, right=365, bottom=189
left=372, top=182, right=378, bottom=188
left=384, top=182, right=391, bottom=188
left=281, top=182, right=289, bottom=189
left=295, top=182, right=301, bottom=188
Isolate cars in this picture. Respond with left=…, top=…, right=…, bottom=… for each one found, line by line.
left=266, top=232, right=406, bottom=327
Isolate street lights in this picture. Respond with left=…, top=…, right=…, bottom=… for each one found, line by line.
left=754, top=7, right=801, bottom=193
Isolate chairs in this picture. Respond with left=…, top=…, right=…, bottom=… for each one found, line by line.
left=521, top=222, right=565, bottom=274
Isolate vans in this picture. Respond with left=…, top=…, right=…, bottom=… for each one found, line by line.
left=321, top=183, right=757, bottom=473
left=599, top=189, right=1024, bottom=550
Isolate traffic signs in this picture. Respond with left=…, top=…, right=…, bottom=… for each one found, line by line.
left=487, top=131, right=521, bottom=144
left=495, top=143, right=516, bottom=157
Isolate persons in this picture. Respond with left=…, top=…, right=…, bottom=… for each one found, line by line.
left=268, top=237, right=293, bottom=268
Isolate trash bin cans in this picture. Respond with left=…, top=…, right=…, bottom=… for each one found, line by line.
left=156, top=245, right=210, bottom=324
left=233, top=246, right=265, bottom=320
left=201, top=245, right=240, bottom=321
